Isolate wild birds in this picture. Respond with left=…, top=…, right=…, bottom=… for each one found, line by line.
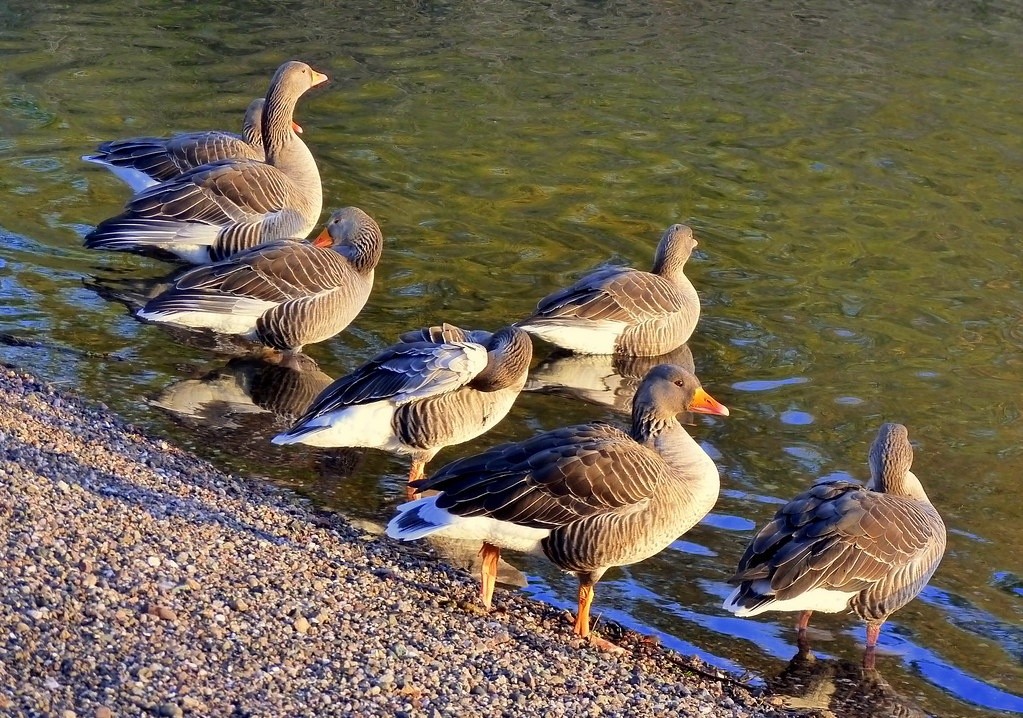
left=511, top=223, right=703, bottom=356
left=270, top=322, right=534, bottom=487
left=385, top=364, right=730, bottom=652
left=720, top=422, right=948, bottom=649
left=83, top=205, right=383, bottom=354
left=81, top=60, right=328, bottom=266
